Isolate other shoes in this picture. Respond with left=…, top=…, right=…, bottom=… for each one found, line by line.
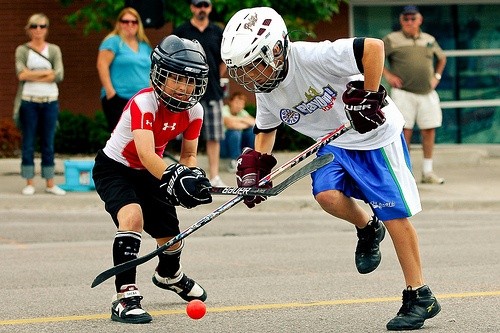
left=205, top=172, right=228, bottom=187
left=45, top=185, right=66, bottom=194
left=22, top=185, right=35, bottom=194
left=230, top=159, right=238, bottom=173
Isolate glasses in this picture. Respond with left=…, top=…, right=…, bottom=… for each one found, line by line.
left=30, top=24, right=47, bottom=28
left=119, top=20, right=138, bottom=24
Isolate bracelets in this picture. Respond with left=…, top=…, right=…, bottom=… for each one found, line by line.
left=435, top=73, right=441, bottom=80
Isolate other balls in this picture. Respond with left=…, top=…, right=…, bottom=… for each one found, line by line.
left=186, top=299, right=206, bottom=320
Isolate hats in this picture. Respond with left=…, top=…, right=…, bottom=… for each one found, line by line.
left=401, top=5, right=417, bottom=15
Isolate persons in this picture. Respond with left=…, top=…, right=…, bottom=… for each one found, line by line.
left=92, top=35, right=212, bottom=324
left=219, top=92, right=256, bottom=173
left=13, top=14, right=66, bottom=195
left=97, top=7, right=154, bottom=133
left=221, top=7, right=441, bottom=331
left=171, top=0, right=227, bottom=187
left=382, top=6, right=445, bottom=184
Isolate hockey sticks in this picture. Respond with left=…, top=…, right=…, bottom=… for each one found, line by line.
left=90, top=98, right=389, bottom=288
left=208, top=151, right=334, bottom=198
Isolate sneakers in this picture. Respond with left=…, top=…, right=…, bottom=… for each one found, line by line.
left=111, top=283, right=153, bottom=324
left=355, top=215, right=386, bottom=274
left=386, top=284, right=442, bottom=330
left=152, top=265, right=208, bottom=303
left=421, top=172, right=444, bottom=185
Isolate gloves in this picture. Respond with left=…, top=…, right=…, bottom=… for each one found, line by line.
left=342, top=81, right=389, bottom=135
left=159, top=163, right=213, bottom=209
left=236, top=147, right=277, bottom=209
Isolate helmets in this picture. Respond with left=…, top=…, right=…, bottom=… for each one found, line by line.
left=192, top=0, right=210, bottom=6
left=220, top=7, right=290, bottom=69
left=149, top=35, right=209, bottom=112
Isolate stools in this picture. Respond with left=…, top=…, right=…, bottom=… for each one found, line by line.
left=63, top=161, right=96, bottom=194
